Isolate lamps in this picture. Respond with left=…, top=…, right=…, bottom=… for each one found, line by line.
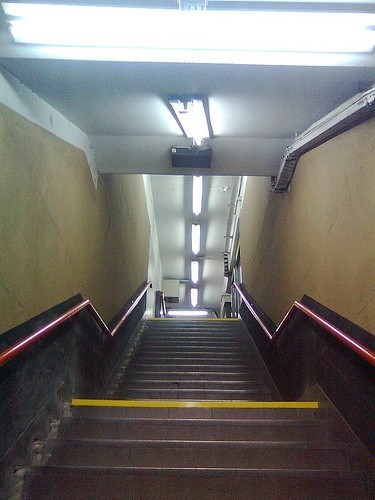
left=168, top=93, right=215, bottom=139
